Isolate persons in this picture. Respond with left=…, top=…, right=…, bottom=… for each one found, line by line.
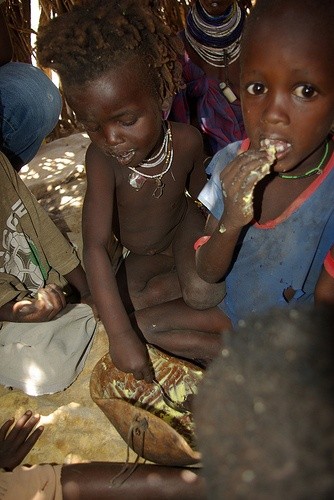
left=36, top=0, right=230, bottom=387
left=0, top=151, right=101, bottom=396
left=126, top=0, right=334, bottom=374
left=0, top=410, right=206, bottom=500
left=166, top=0, right=249, bottom=179
left=0, top=5, right=64, bottom=174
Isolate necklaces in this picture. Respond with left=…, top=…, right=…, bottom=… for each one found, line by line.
left=184, top=0, right=246, bottom=104
left=276, top=139, right=329, bottom=179
left=127, top=122, right=174, bottom=199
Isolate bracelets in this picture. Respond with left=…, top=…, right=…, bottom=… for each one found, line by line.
left=323, top=251, right=334, bottom=279
left=193, top=235, right=212, bottom=250
left=203, top=156, right=213, bottom=164
